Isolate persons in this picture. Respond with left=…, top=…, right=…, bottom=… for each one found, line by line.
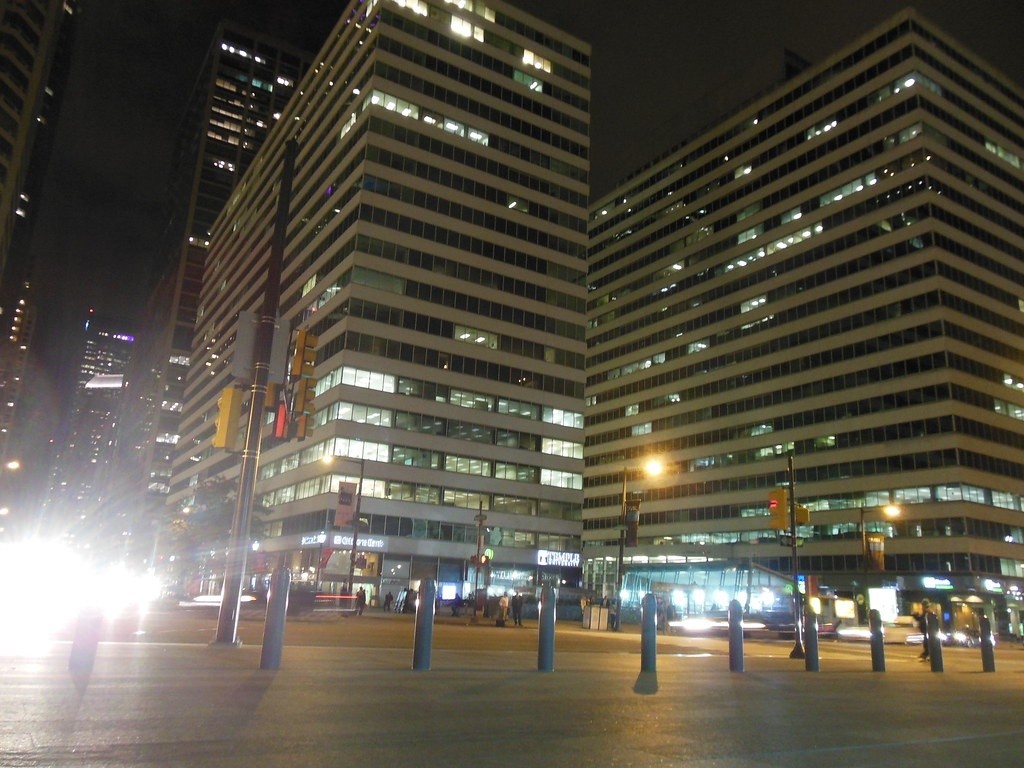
left=499, top=592, right=510, bottom=621
left=910, top=598, right=933, bottom=661
left=512, top=591, right=524, bottom=626
left=354, top=586, right=366, bottom=616
left=383, top=591, right=393, bottom=611
left=68, top=573, right=102, bottom=696
left=451, top=593, right=462, bottom=617
left=586, top=595, right=617, bottom=631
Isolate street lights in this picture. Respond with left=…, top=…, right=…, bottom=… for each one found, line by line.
left=321, top=454, right=366, bottom=609
left=860, top=503, right=901, bottom=588
left=612, top=458, right=665, bottom=632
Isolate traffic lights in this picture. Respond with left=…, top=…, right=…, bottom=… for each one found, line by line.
left=480, top=555, right=492, bottom=566
left=293, top=377, right=318, bottom=413
left=294, top=413, right=315, bottom=442
left=769, top=489, right=787, bottom=529
left=289, top=328, right=320, bottom=382
left=211, top=386, right=244, bottom=451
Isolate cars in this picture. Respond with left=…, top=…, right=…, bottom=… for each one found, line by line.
left=939, top=628, right=997, bottom=648
